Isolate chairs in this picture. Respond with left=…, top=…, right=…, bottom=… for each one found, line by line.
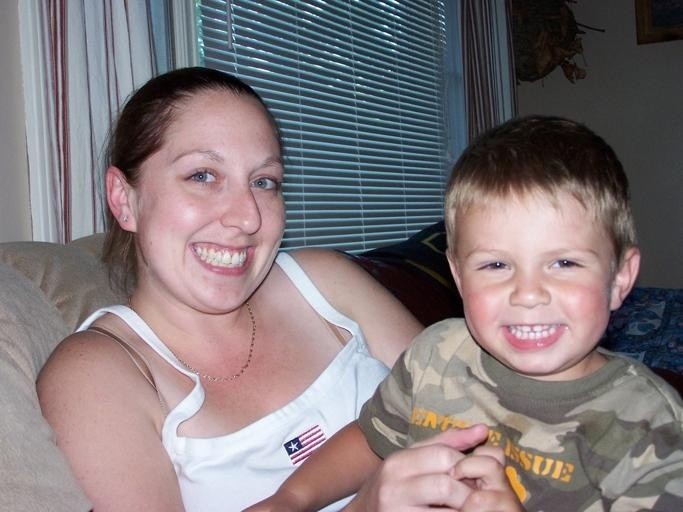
left=0, top=226, right=144, bottom=512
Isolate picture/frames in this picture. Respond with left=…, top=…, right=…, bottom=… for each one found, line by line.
left=633, top=1, right=682, bottom=46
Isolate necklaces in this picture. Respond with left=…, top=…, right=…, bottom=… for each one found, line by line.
left=125, top=296, right=255, bottom=382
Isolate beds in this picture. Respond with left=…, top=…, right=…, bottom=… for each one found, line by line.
left=355, top=217, right=680, bottom=379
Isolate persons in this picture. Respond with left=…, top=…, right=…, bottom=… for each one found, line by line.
left=36, top=66, right=425, bottom=512
left=242, top=113, right=683, bottom=512
